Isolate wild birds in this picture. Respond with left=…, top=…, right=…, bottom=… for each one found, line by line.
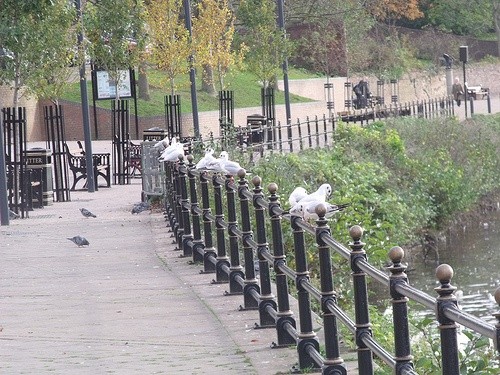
left=218, top=151, right=252, bottom=187
left=208, top=158, right=239, bottom=180
left=67, top=236, right=89, bottom=248
left=288, top=187, right=308, bottom=209
left=303, top=201, right=352, bottom=223
left=131, top=202, right=153, bottom=215
left=79, top=207, right=96, bottom=218
left=153, top=136, right=185, bottom=165
left=188, top=147, right=217, bottom=176
left=280, top=183, right=332, bottom=223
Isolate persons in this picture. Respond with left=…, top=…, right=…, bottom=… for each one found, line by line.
left=356, top=76, right=372, bottom=110
left=451, top=76, right=465, bottom=108
